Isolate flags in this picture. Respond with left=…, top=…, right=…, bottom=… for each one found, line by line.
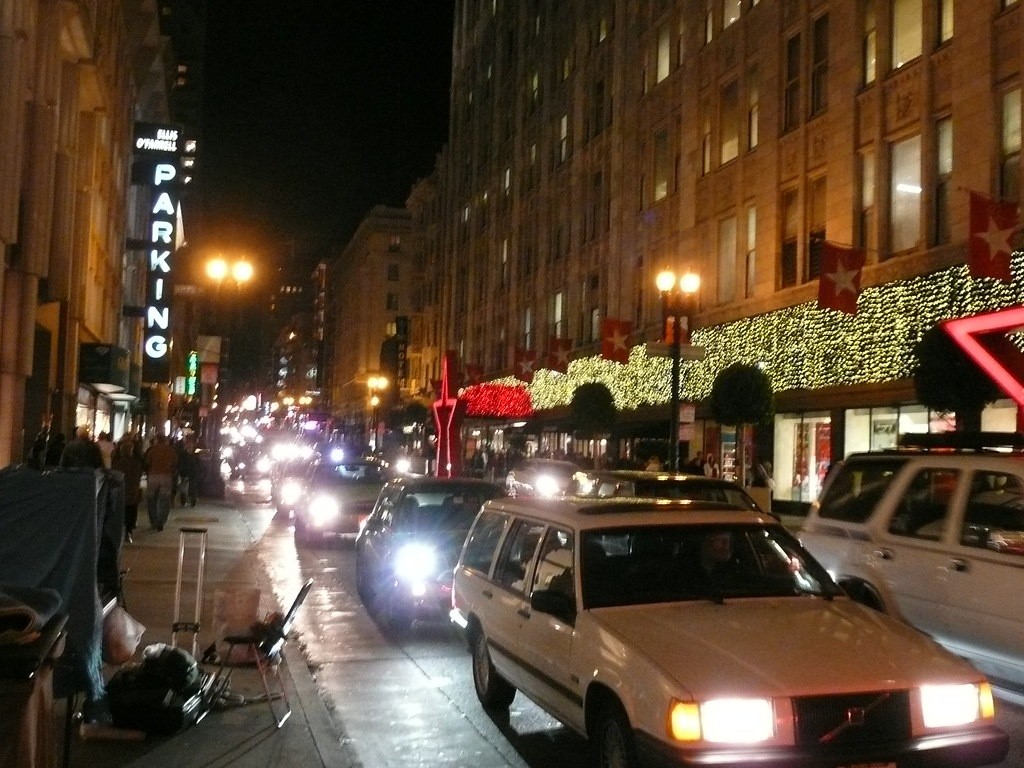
left=968, top=192, right=1017, bottom=279
left=818, top=241, right=866, bottom=314
left=514, top=344, right=536, bottom=384
left=419, top=366, right=481, bottom=402
left=548, top=334, right=573, bottom=374
left=600, top=318, right=632, bottom=364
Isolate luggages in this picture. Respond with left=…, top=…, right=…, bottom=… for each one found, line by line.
left=106, top=529, right=220, bottom=735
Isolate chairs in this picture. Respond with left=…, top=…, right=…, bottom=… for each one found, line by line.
left=572, top=540, right=622, bottom=604
left=200, top=577, right=315, bottom=730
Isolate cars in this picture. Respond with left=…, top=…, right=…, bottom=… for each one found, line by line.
left=565, top=470, right=782, bottom=524
left=256, top=439, right=412, bottom=544
left=446, top=492, right=1009, bottom=768
left=354, top=475, right=516, bottom=632
left=505, top=457, right=583, bottom=497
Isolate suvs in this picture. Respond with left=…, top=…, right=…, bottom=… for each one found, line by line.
left=793, top=429, right=1024, bottom=699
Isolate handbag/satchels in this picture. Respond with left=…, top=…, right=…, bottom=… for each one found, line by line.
left=103, top=594, right=146, bottom=664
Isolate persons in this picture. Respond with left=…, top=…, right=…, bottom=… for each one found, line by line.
left=699, top=531, right=751, bottom=595
left=471, top=446, right=594, bottom=478
left=60, top=432, right=208, bottom=543
left=689, top=451, right=721, bottom=479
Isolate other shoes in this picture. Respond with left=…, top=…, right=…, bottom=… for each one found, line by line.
left=82, top=697, right=104, bottom=723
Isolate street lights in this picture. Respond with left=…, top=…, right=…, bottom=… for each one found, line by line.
left=655, top=263, right=702, bottom=473
left=367, top=376, right=388, bottom=448
left=202, top=254, right=254, bottom=499
